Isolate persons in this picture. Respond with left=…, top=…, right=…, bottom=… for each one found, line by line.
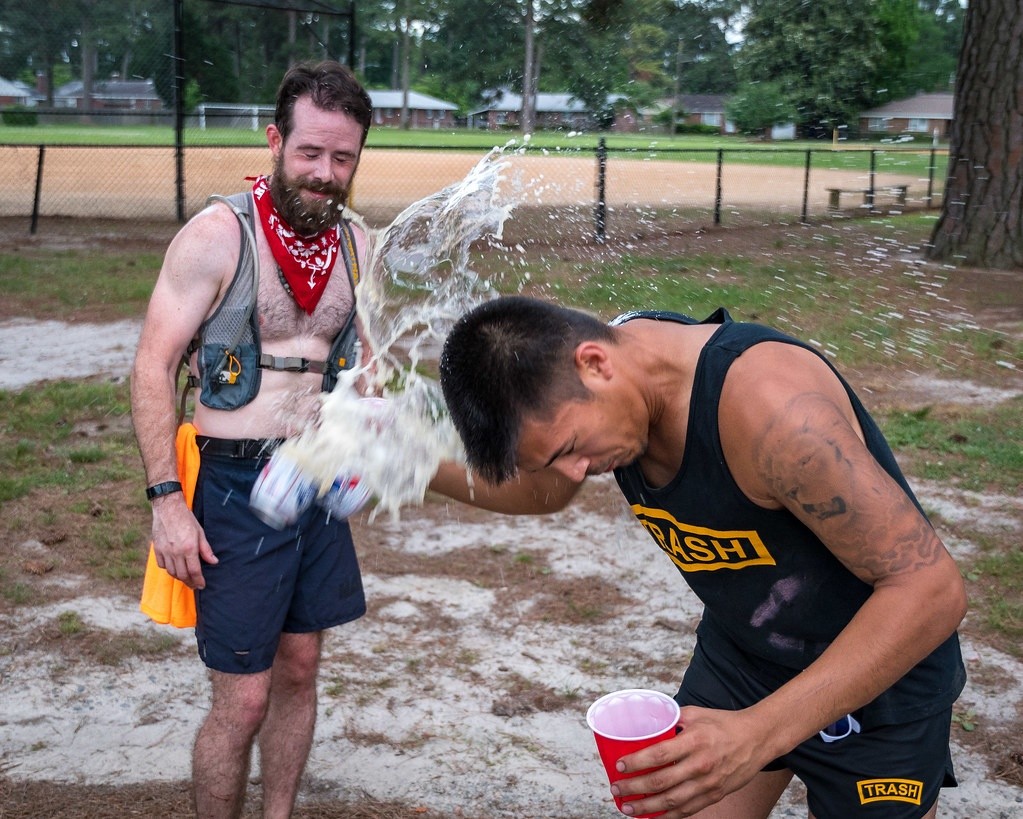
left=130, top=62, right=385, bottom=819
left=285, top=295, right=969, bottom=819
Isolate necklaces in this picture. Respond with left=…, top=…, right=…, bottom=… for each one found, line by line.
left=278, top=265, right=294, bottom=298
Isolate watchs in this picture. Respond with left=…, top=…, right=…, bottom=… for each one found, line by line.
left=146, top=481, right=182, bottom=499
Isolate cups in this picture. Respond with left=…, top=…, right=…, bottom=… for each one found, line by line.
left=587, top=689, right=681, bottom=818
